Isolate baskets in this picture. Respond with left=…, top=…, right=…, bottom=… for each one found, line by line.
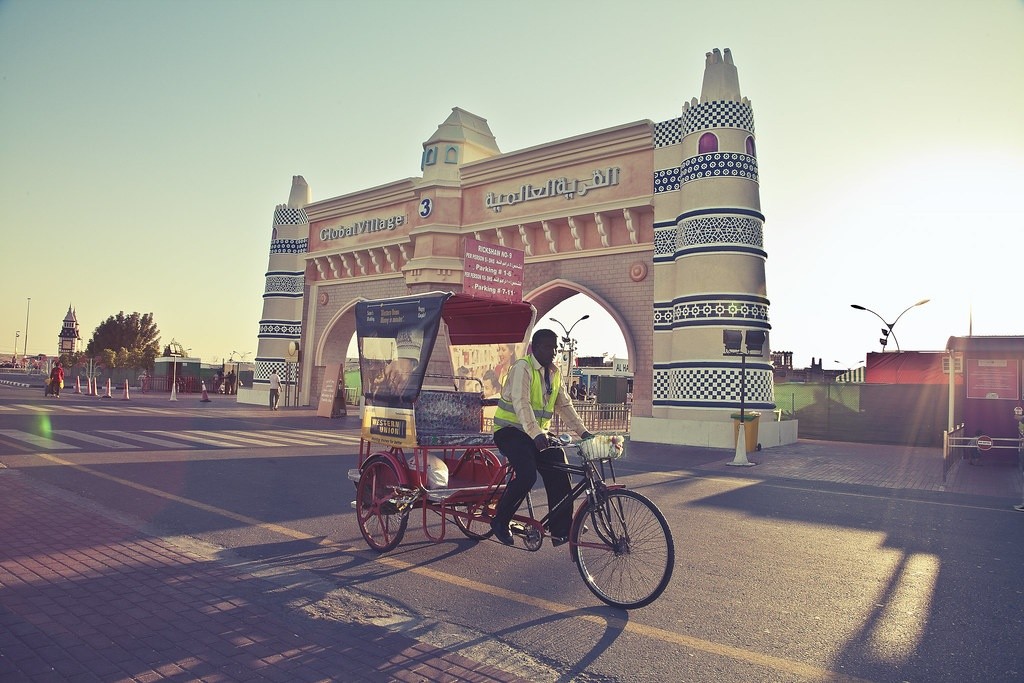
left=575, top=431, right=630, bottom=462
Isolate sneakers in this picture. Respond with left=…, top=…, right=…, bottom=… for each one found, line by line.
left=551, top=525, right=588, bottom=547
left=490, top=518, right=514, bottom=545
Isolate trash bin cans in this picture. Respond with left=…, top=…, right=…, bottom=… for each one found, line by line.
left=730, top=411, right=761, bottom=453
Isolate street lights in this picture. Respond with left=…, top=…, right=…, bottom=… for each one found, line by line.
left=852, top=297, right=929, bottom=352
left=549, top=314, right=589, bottom=389
left=24, top=297, right=32, bottom=356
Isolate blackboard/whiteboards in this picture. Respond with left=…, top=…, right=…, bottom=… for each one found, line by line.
left=317, top=362, right=347, bottom=419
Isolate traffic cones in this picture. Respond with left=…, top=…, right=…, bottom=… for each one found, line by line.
left=120, top=379, right=131, bottom=401
left=73, top=375, right=82, bottom=394
left=84, top=378, right=92, bottom=395
left=101, top=378, right=113, bottom=398
left=89, top=377, right=99, bottom=397
left=199, top=383, right=211, bottom=402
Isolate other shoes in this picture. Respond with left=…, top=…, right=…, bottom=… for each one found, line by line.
left=53, top=394, right=55, bottom=397
left=56, top=395, right=59, bottom=398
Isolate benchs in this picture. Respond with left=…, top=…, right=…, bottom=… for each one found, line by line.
left=390, top=372, right=498, bottom=446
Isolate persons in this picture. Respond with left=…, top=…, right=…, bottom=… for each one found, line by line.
left=490, top=329, right=593, bottom=546
left=570, top=381, right=589, bottom=401
left=50, top=362, right=64, bottom=398
left=213, top=369, right=236, bottom=395
left=269, top=370, right=282, bottom=411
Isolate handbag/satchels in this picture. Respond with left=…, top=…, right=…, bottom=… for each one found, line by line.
left=403, top=454, right=449, bottom=490
left=60, top=380, right=64, bottom=389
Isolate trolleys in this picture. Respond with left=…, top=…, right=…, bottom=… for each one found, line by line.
left=45, top=380, right=61, bottom=397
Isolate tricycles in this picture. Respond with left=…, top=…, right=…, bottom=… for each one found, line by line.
left=341, top=288, right=673, bottom=609
